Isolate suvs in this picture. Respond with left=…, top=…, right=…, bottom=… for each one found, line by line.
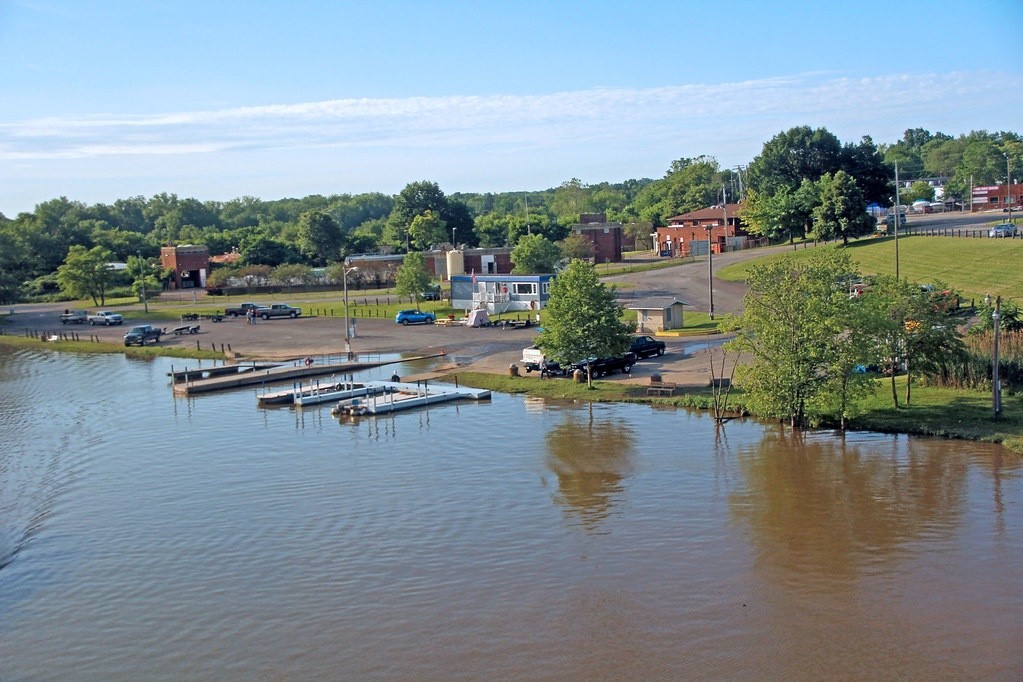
left=922, top=205, right=935, bottom=214
left=881, top=213, right=906, bottom=226
left=395, top=310, right=437, bottom=325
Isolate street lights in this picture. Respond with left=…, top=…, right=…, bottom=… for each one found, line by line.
left=342, top=264, right=359, bottom=351
left=701, top=224, right=713, bottom=320
left=1002, top=152, right=1011, bottom=223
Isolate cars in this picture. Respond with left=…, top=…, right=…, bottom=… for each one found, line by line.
left=989, top=223, right=1018, bottom=238
left=566, top=348, right=639, bottom=379
left=624, top=335, right=667, bottom=361
left=420, top=288, right=440, bottom=301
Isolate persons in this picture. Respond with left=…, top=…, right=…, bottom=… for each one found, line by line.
left=540, top=355, right=550, bottom=379
left=246, top=308, right=257, bottom=326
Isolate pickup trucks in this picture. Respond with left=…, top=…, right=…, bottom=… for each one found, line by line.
left=87, top=311, right=124, bottom=327
left=58, top=309, right=90, bottom=324
left=225, top=303, right=268, bottom=319
left=122, top=325, right=161, bottom=346
left=256, top=303, right=302, bottom=320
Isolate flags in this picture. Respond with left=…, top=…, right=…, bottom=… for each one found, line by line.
left=470, top=271, right=477, bottom=284
